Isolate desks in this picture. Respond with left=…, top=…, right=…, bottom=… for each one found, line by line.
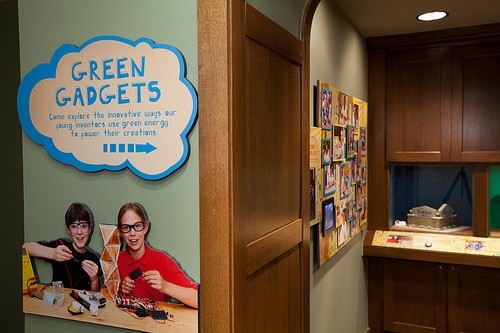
left=23, top=284, right=198, bottom=333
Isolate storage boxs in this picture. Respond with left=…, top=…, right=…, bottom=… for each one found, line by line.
left=407, top=214, right=457, bottom=228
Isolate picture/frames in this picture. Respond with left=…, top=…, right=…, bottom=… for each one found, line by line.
left=333, top=124, right=344, bottom=162
left=321, top=138, right=331, bottom=164
left=322, top=197, right=336, bottom=237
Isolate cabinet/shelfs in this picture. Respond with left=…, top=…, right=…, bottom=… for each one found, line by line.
left=384, top=37, right=500, bottom=163
left=383, top=258, right=500, bottom=333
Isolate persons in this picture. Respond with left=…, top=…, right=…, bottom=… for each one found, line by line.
left=22, top=203, right=103, bottom=292
left=322, top=91, right=366, bottom=223
left=102, top=203, right=198, bottom=308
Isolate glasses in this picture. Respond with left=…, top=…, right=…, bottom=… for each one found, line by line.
left=68, top=221, right=89, bottom=232
left=119, top=222, right=146, bottom=233
